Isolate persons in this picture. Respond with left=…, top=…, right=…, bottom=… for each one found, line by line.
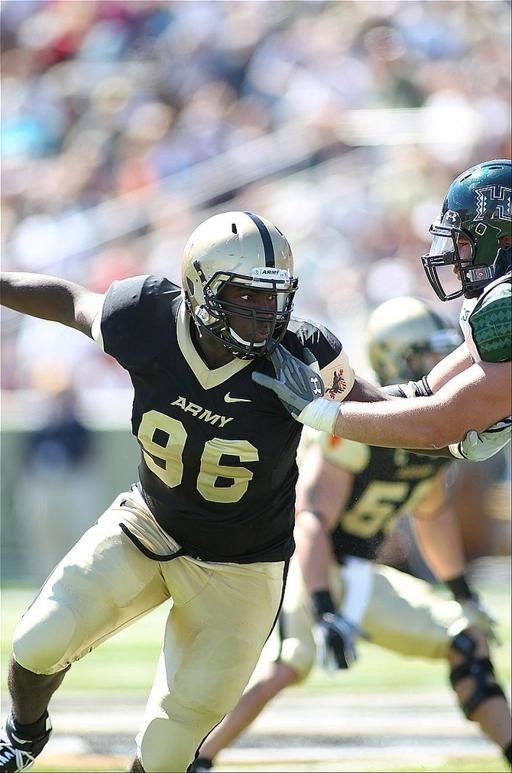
left=0, top=211, right=512, bottom=773
left=251, top=162, right=512, bottom=451
left=186, top=298, right=512, bottom=773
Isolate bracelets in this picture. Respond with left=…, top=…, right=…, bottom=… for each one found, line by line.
left=295, top=397, right=342, bottom=435
left=448, top=443, right=465, bottom=459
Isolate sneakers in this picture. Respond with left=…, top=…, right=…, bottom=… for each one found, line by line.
left=0, top=714, right=54, bottom=772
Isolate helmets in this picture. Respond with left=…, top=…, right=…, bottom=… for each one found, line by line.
left=365, top=296, right=460, bottom=387
left=181, top=209, right=300, bottom=364
left=417, top=157, right=510, bottom=302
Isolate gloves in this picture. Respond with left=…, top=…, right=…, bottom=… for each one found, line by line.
left=443, top=415, right=512, bottom=463
left=249, top=341, right=342, bottom=436
left=455, top=592, right=501, bottom=651
left=309, top=607, right=370, bottom=673
